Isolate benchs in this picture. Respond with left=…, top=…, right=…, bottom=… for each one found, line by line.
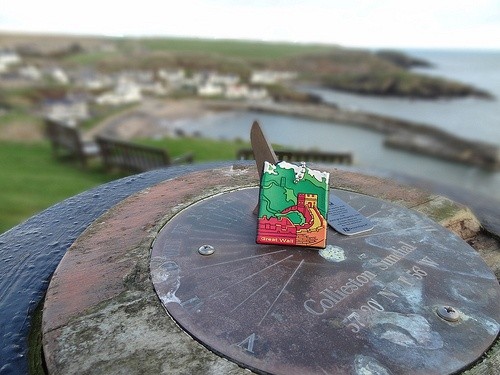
left=91, top=135, right=195, bottom=179
left=43, top=116, right=101, bottom=170
left=235, top=150, right=356, bottom=171
